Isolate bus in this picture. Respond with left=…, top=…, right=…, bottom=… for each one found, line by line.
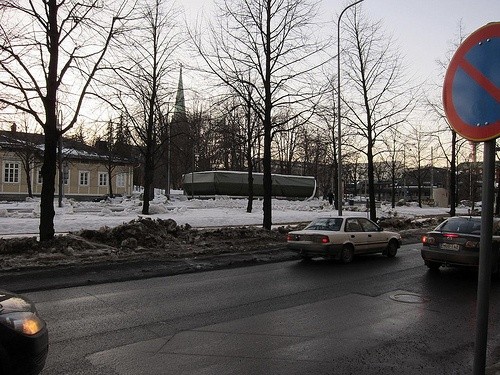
left=395, top=185, right=438, bottom=204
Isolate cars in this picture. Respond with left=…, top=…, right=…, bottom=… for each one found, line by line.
left=285, top=215, right=403, bottom=265
left=420, top=214, right=500, bottom=283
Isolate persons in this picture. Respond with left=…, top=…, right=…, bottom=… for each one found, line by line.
left=326, top=189, right=334, bottom=205
left=333, top=219, right=342, bottom=227
